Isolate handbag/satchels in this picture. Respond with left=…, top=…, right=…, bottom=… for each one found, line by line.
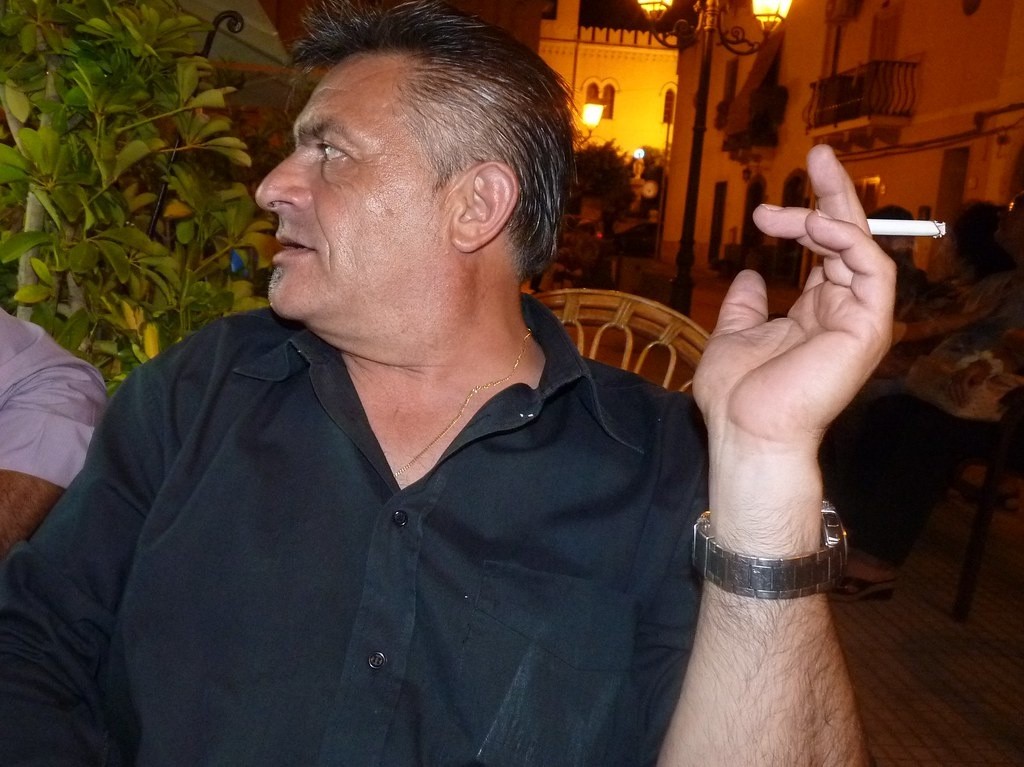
left=903, top=328, right=1024, bottom=422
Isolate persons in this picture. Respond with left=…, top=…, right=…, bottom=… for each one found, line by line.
left=0, top=307, right=108, bottom=560
left=1, top=0, right=898, bottom=766
left=818, top=194, right=1024, bottom=601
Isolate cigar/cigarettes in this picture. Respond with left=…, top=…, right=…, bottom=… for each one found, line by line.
left=866, top=218, right=946, bottom=238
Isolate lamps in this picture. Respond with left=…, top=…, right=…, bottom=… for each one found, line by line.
left=577, top=103, right=605, bottom=148
left=635, top=0, right=793, bottom=57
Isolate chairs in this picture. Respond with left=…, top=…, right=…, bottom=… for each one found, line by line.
left=937, top=377, right=1024, bottom=624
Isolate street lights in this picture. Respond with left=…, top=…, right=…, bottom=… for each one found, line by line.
left=633, top=1, right=793, bottom=319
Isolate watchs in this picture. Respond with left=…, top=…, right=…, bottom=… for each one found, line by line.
left=692, top=497, right=848, bottom=599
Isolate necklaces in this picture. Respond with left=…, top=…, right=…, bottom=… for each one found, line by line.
left=394, top=328, right=532, bottom=476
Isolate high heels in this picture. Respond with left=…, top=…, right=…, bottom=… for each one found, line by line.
left=832, top=575, right=897, bottom=602
